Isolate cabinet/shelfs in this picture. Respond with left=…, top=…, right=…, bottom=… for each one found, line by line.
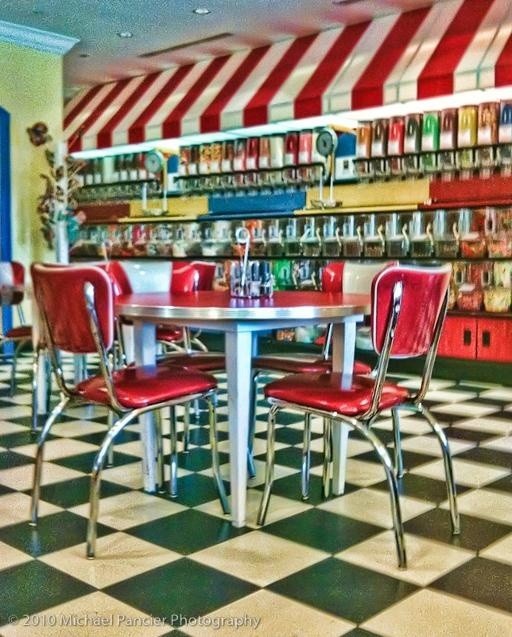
left=64, top=84, right=512, bottom=382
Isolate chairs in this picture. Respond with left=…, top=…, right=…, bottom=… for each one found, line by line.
left=256, top=263, right=460, bottom=572
left=248, top=260, right=404, bottom=480
left=0, top=261, right=33, bottom=397
left=29, top=261, right=231, bottom=559
